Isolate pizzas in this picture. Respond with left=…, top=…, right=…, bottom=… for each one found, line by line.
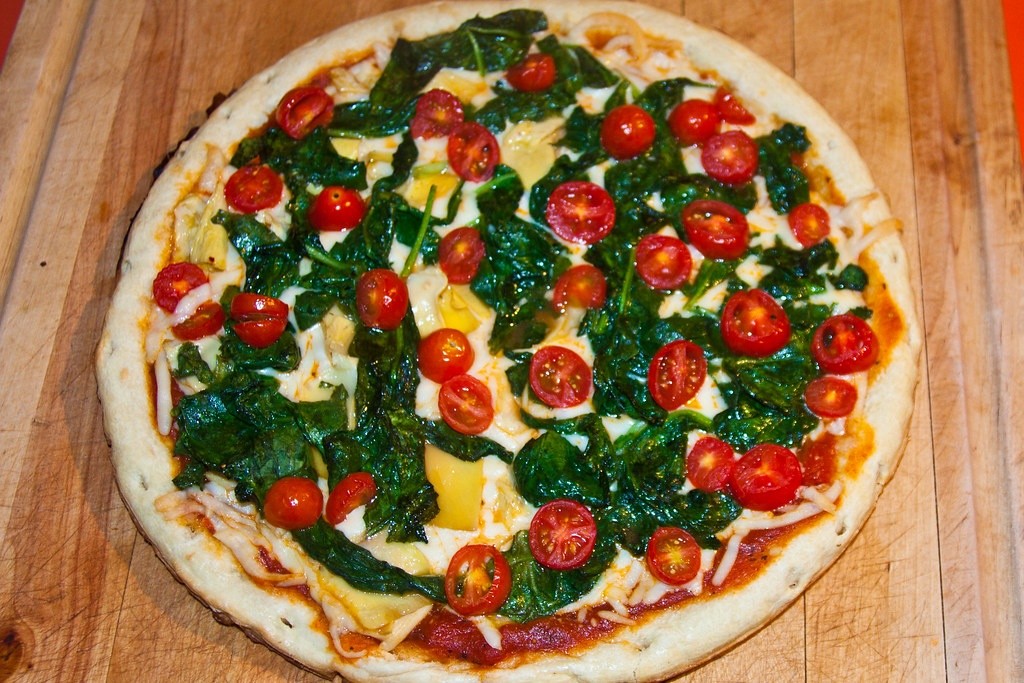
left=96, top=0, right=923, bottom=683
